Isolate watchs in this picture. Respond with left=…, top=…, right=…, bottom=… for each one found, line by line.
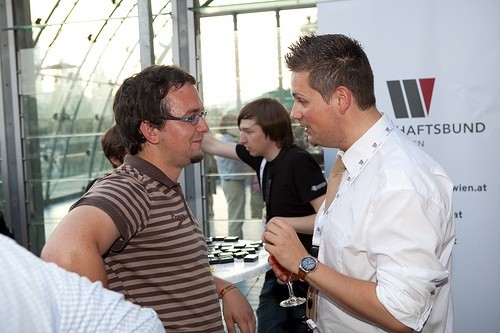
left=299, top=255, right=320, bottom=280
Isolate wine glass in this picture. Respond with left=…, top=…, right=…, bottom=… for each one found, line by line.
left=270, top=253, right=306, bottom=307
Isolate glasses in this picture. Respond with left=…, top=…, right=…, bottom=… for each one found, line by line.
left=160, top=111, right=208, bottom=125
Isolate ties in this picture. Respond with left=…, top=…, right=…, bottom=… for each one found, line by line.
left=306, top=154, right=347, bottom=322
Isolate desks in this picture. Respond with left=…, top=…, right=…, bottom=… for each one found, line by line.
left=207, top=241, right=272, bottom=297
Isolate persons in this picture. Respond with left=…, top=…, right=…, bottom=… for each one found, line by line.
left=200, top=97, right=328, bottom=333
left=212, top=108, right=250, bottom=240
left=262, top=32, right=456, bottom=333
left=0, top=233, right=166, bottom=333
left=37, top=65, right=256, bottom=333
left=85, top=123, right=127, bottom=191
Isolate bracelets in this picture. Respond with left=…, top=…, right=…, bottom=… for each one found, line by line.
left=220, top=284, right=237, bottom=299
